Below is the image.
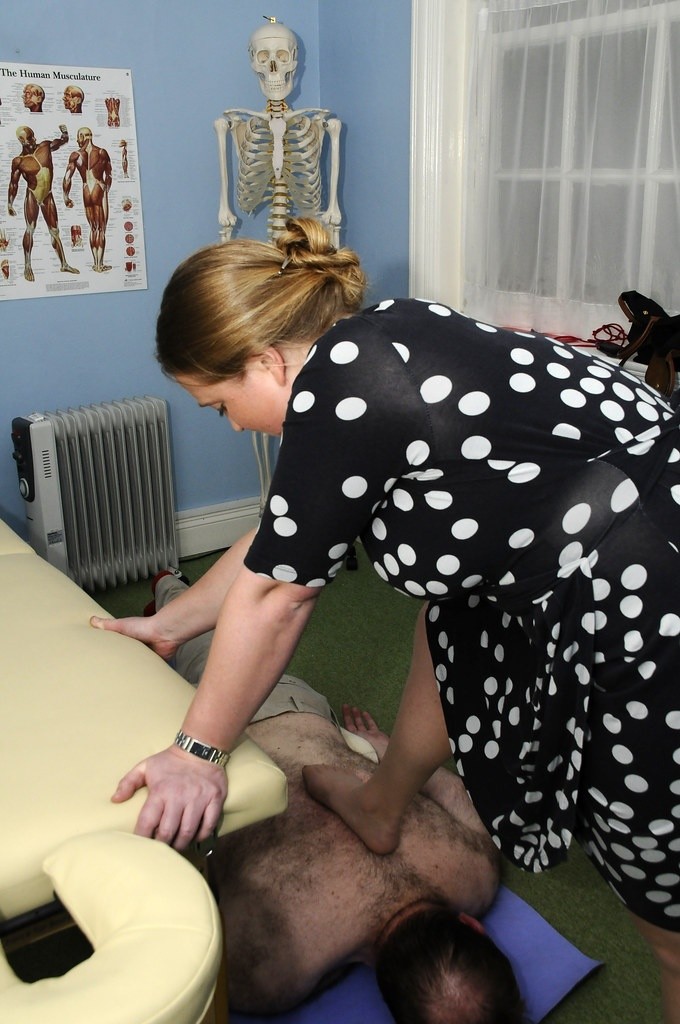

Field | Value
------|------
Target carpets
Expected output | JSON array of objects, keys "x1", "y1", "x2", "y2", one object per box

[{"x1": 227, "y1": 881, "x2": 603, "y2": 1024}]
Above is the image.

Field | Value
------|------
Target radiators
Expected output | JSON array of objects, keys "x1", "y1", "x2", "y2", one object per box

[{"x1": 11, "y1": 397, "x2": 184, "y2": 594}]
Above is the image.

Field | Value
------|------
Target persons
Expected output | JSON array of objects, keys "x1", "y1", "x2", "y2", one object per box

[{"x1": 96, "y1": 213, "x2": 679, "y2": 1021}]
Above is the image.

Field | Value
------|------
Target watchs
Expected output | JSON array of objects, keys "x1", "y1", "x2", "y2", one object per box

[{"x1": 174, "y1": 731, "x2": 231, "y2": 768}]
